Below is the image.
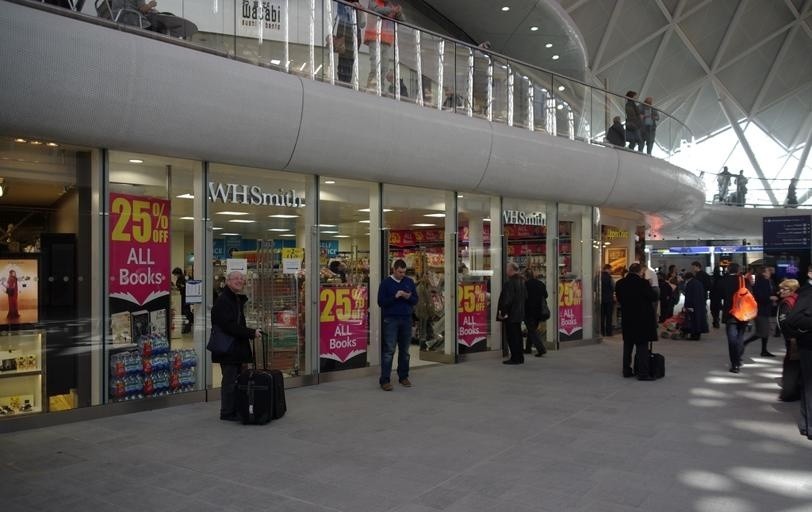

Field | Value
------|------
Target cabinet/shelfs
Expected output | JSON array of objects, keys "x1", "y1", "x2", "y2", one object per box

[
  {"x1": 225, "y1": 238, "x2": 574, "y2": 379},
  {"x1": 0, "y1": 328, "x2": 48, "y2": 418}
]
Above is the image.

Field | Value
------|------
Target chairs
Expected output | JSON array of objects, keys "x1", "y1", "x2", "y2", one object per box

[{"x1": 95, "y1": 0, "x2": 143, "y2": 29}]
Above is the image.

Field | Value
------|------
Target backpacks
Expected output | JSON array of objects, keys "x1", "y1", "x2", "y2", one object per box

[{"x1": 728, "y1": 275, "x2": 760, "y2": 322}]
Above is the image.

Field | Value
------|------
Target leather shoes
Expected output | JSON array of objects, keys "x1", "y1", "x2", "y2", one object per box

[
  {"x1": 686, "y1": 337, "x2": 700, "y2": 340},
  {"x1": 729, "y1": 365, "x2": 740, "y2": 373},
  {"x1": 762, "y1": 352, "x2": 774, "y2": 356},
  {"x1": 503, "y1": 348, "x2": 546, "y2": 364},
  {"x1": 624, "y1": 373, "x2": 634, "y2": 377},
  {"x1": 639, "y1": 375, "x2": 656, "y2": 381}
]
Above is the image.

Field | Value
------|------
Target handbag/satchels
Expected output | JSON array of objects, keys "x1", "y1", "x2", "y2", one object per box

[
  {"x1": 7, "y1": 288, "x2": 14, "y2": 295},
  {"x1": 682, "y1": 311, "x2": 697, "y2": 334},
  {"x1": 627, "y1": 119, "x2": 640, "y2": 130},
  {"x1": 540, "y1": 296, "x2": 551, "y2": 322},
  {"x1": 333, "y1": 36, "x2": 346, "y2": 54},
  {"x1": 207, "y1": 329, "x2": 237, "y2": 357},
  {"x1": 633, "y1": 350, "x2": 665, "y2": 378}
]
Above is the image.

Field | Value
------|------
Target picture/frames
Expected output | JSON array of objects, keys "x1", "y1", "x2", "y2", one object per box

[{"x1": 0, "y1": 251, "x2": 43, "y2": 331}]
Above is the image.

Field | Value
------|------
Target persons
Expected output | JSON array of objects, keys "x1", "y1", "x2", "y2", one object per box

[
  {"x1": 171, "y1": 268, "x2": 193, "y2": 334},
  {"x1": 6, "y1": 269, "x2": 20, "y2": 319},
  {"x1": 337, "y1": 0, "x2": 409, "y2": 101},
  {"x1": 522, "y1": 268, "x2": 548, "y2": 357},
  {"x1": 442, "y1": 84, "x2": 466, "y2": 110},
  {"x1": 496, "y1": 261, "x2": 528, "y2": 365},
  {"x1": 784, "y1": 178, "x2": 798, "y2": 208},
  {"x1": 716, "y1": 166, "x2": 738, "y2": 201},
  {"x1": 206, "y1": 270, "x2": 262, "y2": 422},
  {"x1": 377, "y1": 260, "x2": 419, "y2": 391},
  {"x1": 607, "y1": 90, "x2": 659, "y2": 154},
  {"x1": 733, "y1": 170, "x2": 748, "y2": 207},
  {"x1": 112, "y1": 0, "x2": 168, "y2": 34},
  {"x1": 329, "y1": 260, "x2": 346, "y2": 283},
  {"x1": 594, "y1": 262, "x2": 812, "y2": 440}
]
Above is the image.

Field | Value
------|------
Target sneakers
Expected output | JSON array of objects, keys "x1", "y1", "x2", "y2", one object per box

[
  {"x1": 381, "y1": 383, "x2": 393, "y2": 391},
  {"x1": 220, "y1": 413, "x2": 236, "y2": 420},
  {"x1": 399, "y1": 379, "x2": 412, "y2": 387}
]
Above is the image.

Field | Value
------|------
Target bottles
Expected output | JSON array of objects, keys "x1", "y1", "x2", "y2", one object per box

[{"x1": 110, "y1": 332, "x2": 197, "y2": 401}]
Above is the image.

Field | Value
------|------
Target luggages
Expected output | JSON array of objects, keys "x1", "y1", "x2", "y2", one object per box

[
  {"x1": 264, "y1": 333, "x2": 287, "y2": 419},
  {"x1": 235, "y1": 331, "x2": 272, "y2": 426}
]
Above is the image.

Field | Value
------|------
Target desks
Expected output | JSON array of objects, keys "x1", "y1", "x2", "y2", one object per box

[{"x1": 148, "y1": 13, "x2": 198, "y2": 39}]
